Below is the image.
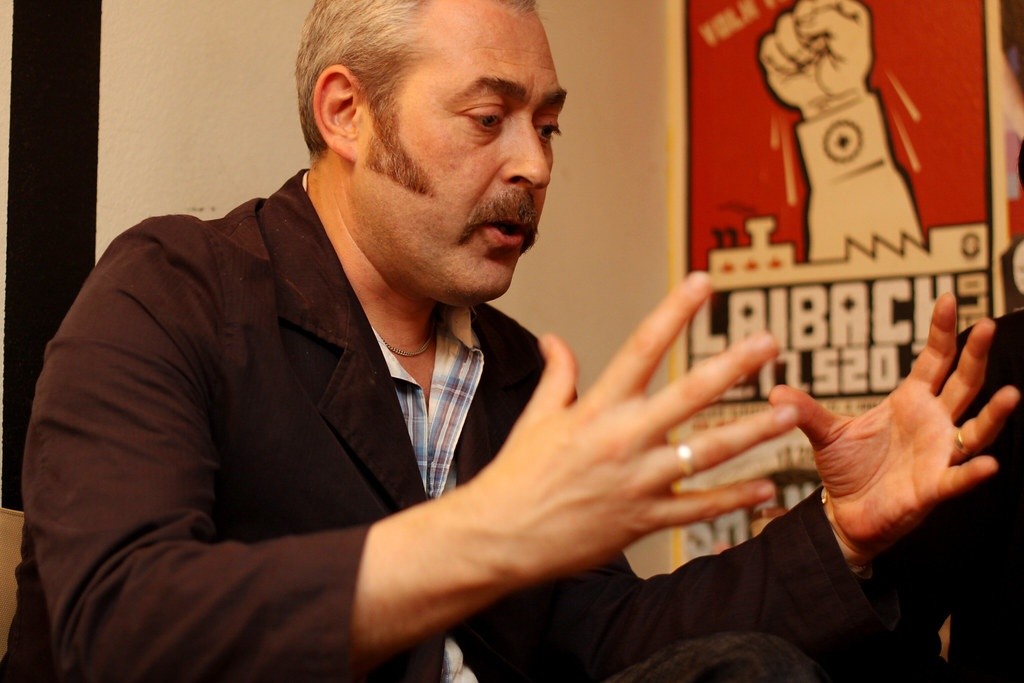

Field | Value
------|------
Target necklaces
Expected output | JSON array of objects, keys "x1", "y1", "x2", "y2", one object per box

[{"x1": 383, "y1": 320, "x2": 437, "y2": 355}]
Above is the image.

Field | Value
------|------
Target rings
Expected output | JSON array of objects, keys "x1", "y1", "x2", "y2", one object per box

[
  {"x1": 678, "y1": 443, "x2": 693, "y2": 477},
  {"x1": 956, "y1": 430, "x2": 970, "y2": 453}
]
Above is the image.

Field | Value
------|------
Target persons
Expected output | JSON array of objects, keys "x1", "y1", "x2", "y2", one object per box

[
  {"x1": 818, "y1": 145, "x2": 1024, "y2": 682},
  {"x1": 0, "y1": 0, "x2": 1019, "y2": 683}
]
